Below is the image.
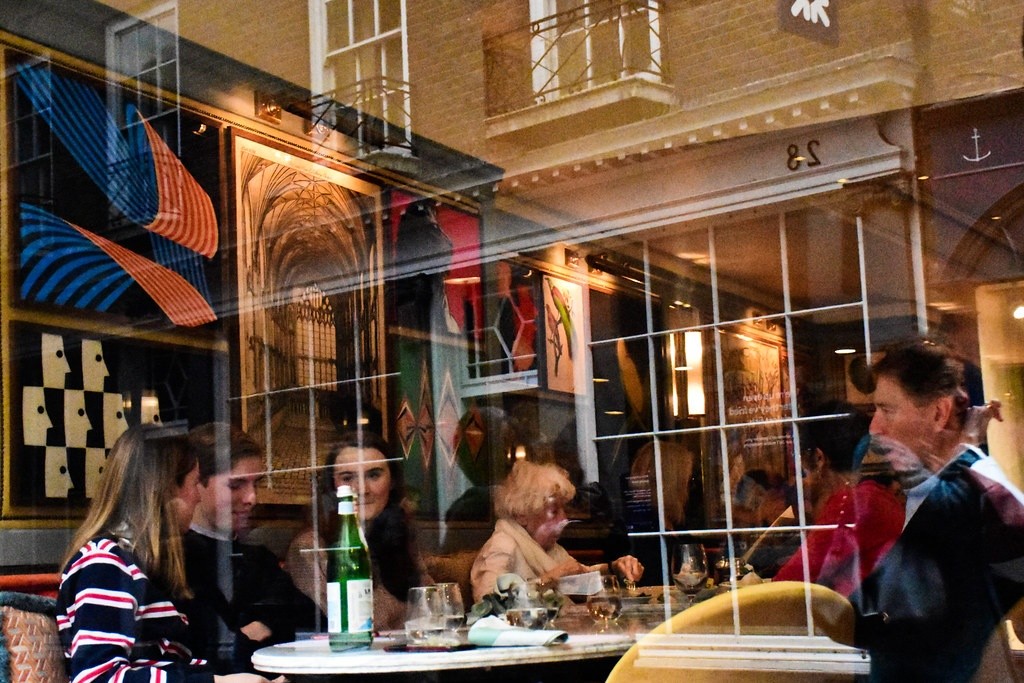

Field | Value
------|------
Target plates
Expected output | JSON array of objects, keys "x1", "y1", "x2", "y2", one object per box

[
  {"x1": 719, "y1": 581, "x2": 745, "y2": 587},
  {"x1": 621, "y1": 596, "x2": 653, "y2": 605}
]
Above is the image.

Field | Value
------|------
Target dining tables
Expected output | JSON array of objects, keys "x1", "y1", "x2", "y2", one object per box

[{"x1": 250, "y1": 630, "x2": 635, "y2": 683}]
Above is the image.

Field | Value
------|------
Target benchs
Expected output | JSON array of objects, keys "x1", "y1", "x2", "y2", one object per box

[{"x1": 1, "y1": 550, "x2": 605, "y2": 600}]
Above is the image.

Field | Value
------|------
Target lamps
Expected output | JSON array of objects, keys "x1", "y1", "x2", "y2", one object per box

[{"x1": 675, "y1": 330, "x2": 706, "y2": 417}]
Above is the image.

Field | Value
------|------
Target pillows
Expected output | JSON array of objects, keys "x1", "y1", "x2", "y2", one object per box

[
  {"x1": 0, "y1": 590, "x2": 68, "y2": 683},
  {"x1": 428, "y1": 553, "x2": 478, "y2": 613}
]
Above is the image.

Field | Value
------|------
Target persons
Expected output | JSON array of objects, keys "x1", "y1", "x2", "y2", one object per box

[
  {"x1": 52, "y1": 422, "x2": 287, "y2": 683},
  {"x1": 738, "y1": 395, "x2": 924, "y2": 607},
  {"x1": 469, "y1": 460, "x2": 644, "y2": 606},
  {"x1": 869, "y1": 472, "x2": 907, "y2": 513},
  {"x1": 175, "y1": 423, "x2": 339, "y2": 683},
  {"x1": 831, "y1": 341, "x2": 1023, "y2": 683},
  {"x1": 285, "y1": 427, "x2": 466, "y2": 639}
]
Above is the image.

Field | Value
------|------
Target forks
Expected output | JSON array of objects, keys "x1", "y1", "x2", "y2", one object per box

[{"x1": 626, "y1": 580, "x2": 635, "y2": 591}]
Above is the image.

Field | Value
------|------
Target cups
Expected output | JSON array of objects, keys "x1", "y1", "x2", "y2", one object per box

[
  {"x1": 587, "y1": 576, "x2": 622, "y2": 622},
  {"x1": 505, "y1": 583, "x2": 546, "y2": 630},
  {"x1": 405, "y1": 586, "x2": 447, "y2": 648},
  {"x1": 543, "y1": 588, "x2": 559, "y2": 628},
  {"x1": 724, "y1": 558, "x2": 744, "y2": 581},
  {"x1": 434, "y1": 582, "x2": 464, "y2": 647}
]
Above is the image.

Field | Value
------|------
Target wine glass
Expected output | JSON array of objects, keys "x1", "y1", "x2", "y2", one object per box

[{"x1": 671, "y1": 544, "x2": 708, "y2": 607}]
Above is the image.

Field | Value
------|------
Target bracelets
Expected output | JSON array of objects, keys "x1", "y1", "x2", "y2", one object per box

[{"x1": 607, "y1": 562, "x2": 615, "y2": 575}]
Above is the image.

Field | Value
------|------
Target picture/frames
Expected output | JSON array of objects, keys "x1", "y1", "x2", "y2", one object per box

[
  {"x1": 9, "y1": 319, "x2": 226, "y2": 506},
  {"x1": 227, "y1": 126, "x2": 398, "y2": 521},
  {"x1": 540, "y1": 271, "x2": 586, "y2": 398},
  {"x1": 496, "y1": 258, "x2": 545, "y2": 391},
  {"x1": 390, "y1": 186, "x2": 488, "y2": 342},
  {"x1": 587, "y1": 283, "x2": 668, "y2": 524},
  {"x1": 700, "y1": 328, "x2": 789, "y2": 527},
  {"x1": 390, "y1": 333, "x2": 495, "y2": 530},
  {"x1": 3, "y1": 48, "x2": 227, "y2": 340}
]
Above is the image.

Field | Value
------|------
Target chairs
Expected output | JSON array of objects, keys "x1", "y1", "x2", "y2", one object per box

[{"x1": 605, "y1": 581, "x2": 856, "y2": 683}]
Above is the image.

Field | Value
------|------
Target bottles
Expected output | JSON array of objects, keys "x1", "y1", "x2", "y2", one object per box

[
  {"x1": 335, "y1": 484, "x2": 373, "y2": 581},
  {"x1": 327, "y1": 501, "x2": 374, "y2": 652}
]
